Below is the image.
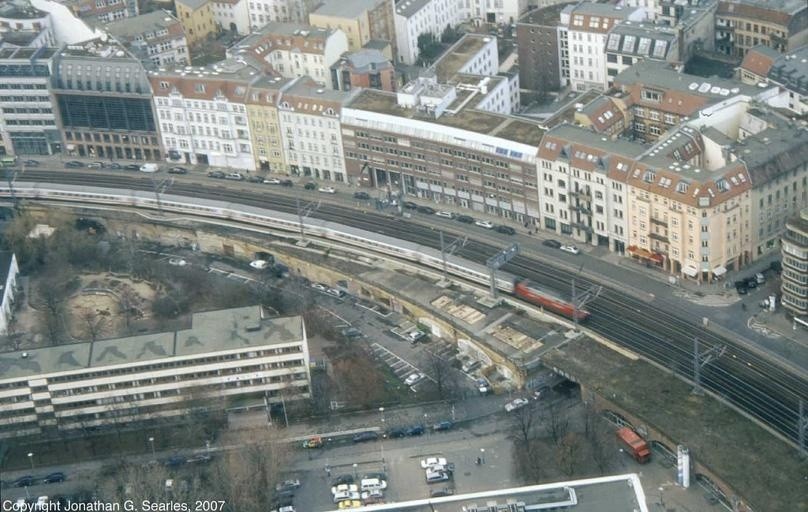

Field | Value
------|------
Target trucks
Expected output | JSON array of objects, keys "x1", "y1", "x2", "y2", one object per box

[{"x1": 617, "y1": 426, "x2": 650, "y2": 464}]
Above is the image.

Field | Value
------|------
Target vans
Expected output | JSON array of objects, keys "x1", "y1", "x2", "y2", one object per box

[
  {"x1": 302, "y1": 437, "x2": 322, "y2": 449},
  {"x1": 1, "y1": 157, "x2": 16, "y2": 167},
  {"x1": 248, "y1": 259, "x2": 346, "y2": 298},
  {"x1": 407, "y1": 330, "x2": 426, "y2": 343},
  {"x1": 734, "y1": 272, "x2": 766, "y2": 294}
]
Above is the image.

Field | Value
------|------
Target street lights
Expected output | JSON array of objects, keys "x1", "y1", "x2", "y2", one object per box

[
  {"x1": 27, "y1": 452, "x2": 34, "y2": 469},
  {"x1": 149, "y1": 437, "x2": 156, "y2": 456}
]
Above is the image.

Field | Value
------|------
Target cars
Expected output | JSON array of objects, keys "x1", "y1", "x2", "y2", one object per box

[
  {"x1": 354, "y1": 191, "x2": 515, "y2": 236},
  {"x1": 167, "y1": 166, "x2": 187, "y2": 174},
  {"x1": 462, "y1": 357, "x2": 492, "y2": 395},
  {"x1": 304, "y1": 180, "x2": 336, "y2": 194},
  {"x1": 421, "y1": 457, "x2": 453, "y2": 497},
  {"x1": 542, "y1": 239, "x2": 579, "y2": 255},
  {"x1": 0, "y1": 453, "x2": 213, "y2": 512},
  {"x1": 208, "y1": 170, "x2": 292, "y2": 186},
  {"x1": 505, "y1": 386, "x2": 549, "y2": 413},
  {"x1": 351, "y1": 420, "x2": 453, "y2": 443},
  {"x1": 64, "y1": 160, "x2": 159, "y2": 173},
  {"x1": 330, "y1": 472, "x2": 388, "y2": 509},
  {"x1": 405, "y1": 372, "x2": 425, "y2": 385},
  {"x1": 23, "y1": 160, "x2": 39, "y2": 167},
  {"x1": 269, "y1": 477, "x2": 300, "y2": 512}
]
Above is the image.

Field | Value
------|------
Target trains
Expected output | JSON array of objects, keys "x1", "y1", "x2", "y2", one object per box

[{"x1": 0, "y1": 180, "x2": 590, "y2": 322}]
